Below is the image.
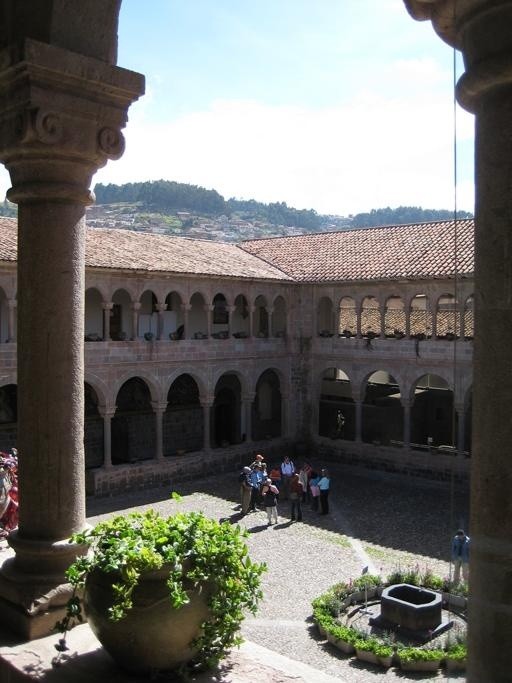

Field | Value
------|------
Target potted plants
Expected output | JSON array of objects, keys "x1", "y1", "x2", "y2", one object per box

[
  {"x1": 310, "y1": 568, "x2": 468, "y2": 672},
  {"x1": 48, "y1": 492, "x2": 270, "y2": 683}
]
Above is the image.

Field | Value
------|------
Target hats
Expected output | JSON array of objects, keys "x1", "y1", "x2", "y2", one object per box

[{"x1": 256, "y1": 455, "x2": 264, "y2": 460}]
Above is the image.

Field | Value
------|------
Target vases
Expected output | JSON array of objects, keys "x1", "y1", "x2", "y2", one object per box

[{"x1": 0, "y1": 465, "x2": 12, "y2": 519}]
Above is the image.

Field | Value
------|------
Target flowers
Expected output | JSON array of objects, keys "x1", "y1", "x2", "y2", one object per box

[{"x1": 0, "y1": 447, "x2": 20, "y2": 538}]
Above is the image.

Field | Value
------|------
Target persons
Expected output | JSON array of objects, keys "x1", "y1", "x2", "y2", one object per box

[
  {"x1": 452, "y1": 530, "x2": 470, "y2": 583},
  {"x1": 337, "y1": 409, "x2": 345, "y2": 439},
  {"x1": 240, "y1": 453, "x2": 329, "y2": 525}
]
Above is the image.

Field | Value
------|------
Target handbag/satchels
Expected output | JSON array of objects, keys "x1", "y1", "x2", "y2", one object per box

[
  {"x1": 290, "y1": 492, "x2": 297, "y2": 500},
  {"x1": 310, "y1": 485, "x2": 320, "y2": 497}
]
